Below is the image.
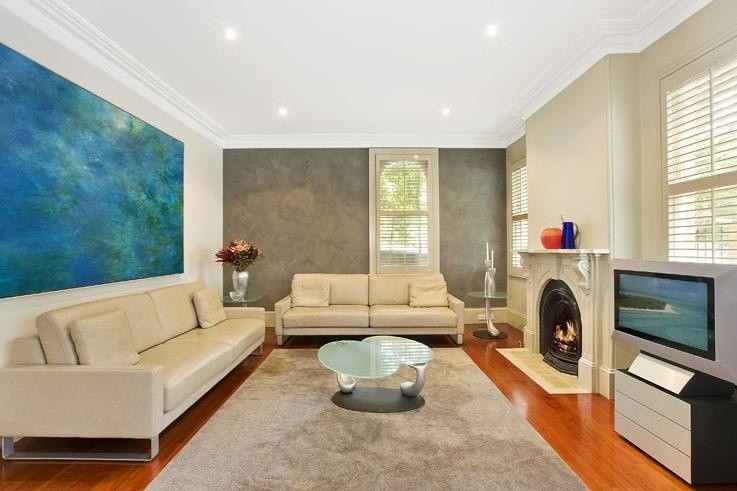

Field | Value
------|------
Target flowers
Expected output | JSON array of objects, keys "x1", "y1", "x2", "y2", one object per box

[{"x1": 213, "y1": 237, "x2": 262, "y2": 270}]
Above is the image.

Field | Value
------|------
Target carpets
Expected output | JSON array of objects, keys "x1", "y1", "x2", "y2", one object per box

[{"x1": 142, "y1": 343, "x2": 589, "y2": 490}]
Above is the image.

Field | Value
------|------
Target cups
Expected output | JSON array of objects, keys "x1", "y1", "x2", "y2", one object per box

[
  {"x1": 484, "y1": 260, "x2": 496, "y2": 298},
  {"x1": 560, "y1": 222, "x2": 578, "y2": 249},
  {"x1": 229, "y1": 292, "x2": 242, "y2": 301}
]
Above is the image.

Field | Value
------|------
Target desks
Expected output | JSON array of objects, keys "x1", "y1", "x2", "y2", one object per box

[{"x1": 608, "y1": 368, "x2": 736, "y2": 486}]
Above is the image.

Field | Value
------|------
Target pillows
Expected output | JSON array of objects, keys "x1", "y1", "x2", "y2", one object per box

[
  {"x1": 288, "y1": 276, "x2": 331, "y2": 309},
  {"x1": 408, "y1": 279, "x2": 448, "y2": 309},
  {"x1": 191, "y1": 287, "x2": 226, "y2": 330},
  {"x1": 68, "y1": 310, "x2": 139, "y2": 371}
]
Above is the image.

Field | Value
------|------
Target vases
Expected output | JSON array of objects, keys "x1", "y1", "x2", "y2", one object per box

[
  {"x1": 229, "y1": 269, "x2": 248, "y2": 298},
  {"x1": 540, "y1": 228, "x2": 562, "y2": 250}
]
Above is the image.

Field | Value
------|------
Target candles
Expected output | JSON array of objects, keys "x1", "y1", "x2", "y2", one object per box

[
  {"x1": 491, "y1": 250, "x2": 493, "y2": 270},
  {"x1": 486, "y1": 242, "x2": 488, "y2": 261}
]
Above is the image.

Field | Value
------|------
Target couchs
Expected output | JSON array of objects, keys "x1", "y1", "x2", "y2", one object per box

[
  {"x1": 269, "y1": 273, "x2": 465, "y2": 349},
  {"x1": 0, "y1": 271, "x2": 267, "y2": 466}
]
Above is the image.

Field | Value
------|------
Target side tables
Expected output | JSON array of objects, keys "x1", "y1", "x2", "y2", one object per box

[
  {"x1": 218, "y1": 293, "x2": 266, "y2": 308},
  {"x1": 467, "y1": 289, "x2": 514, "y2": 340}
]
